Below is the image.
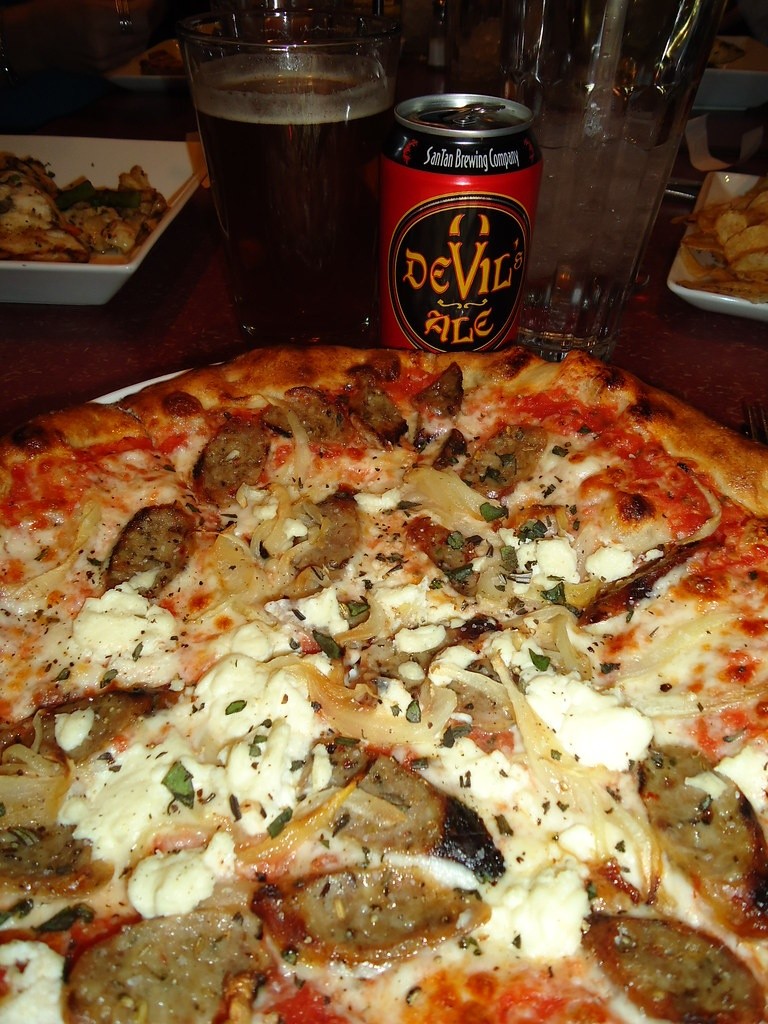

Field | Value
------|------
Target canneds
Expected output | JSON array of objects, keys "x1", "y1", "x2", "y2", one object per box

[{"x1": 382, "y1": 93, "x2": 540, "y2": 347}]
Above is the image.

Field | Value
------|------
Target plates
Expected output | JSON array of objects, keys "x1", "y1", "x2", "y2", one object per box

[
  {"x1": 0, "y1": 134, "x2": 213, "y2": 306},
  {"x1": 666, "y1": 171, "x2": 768, "y2": 322},
  {"x1": 693, "y1": 35, "x2": 768, "y2": 110},
  {"x1": 102, "y1": 38, "x2": 189, "y2": 93}
]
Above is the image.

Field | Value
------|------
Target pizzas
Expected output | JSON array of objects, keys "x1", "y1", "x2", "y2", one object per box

[{"x1": 0, "y1": 349, "x2": 768, "y2": 1024}]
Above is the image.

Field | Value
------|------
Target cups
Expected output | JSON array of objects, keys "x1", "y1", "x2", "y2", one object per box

[
  {"x1": 173, "y1": 8, "x2": 402, "y2": 351},
  {"x1": 497, "y1": 0, "x2": 725, "y2": 367}
]
